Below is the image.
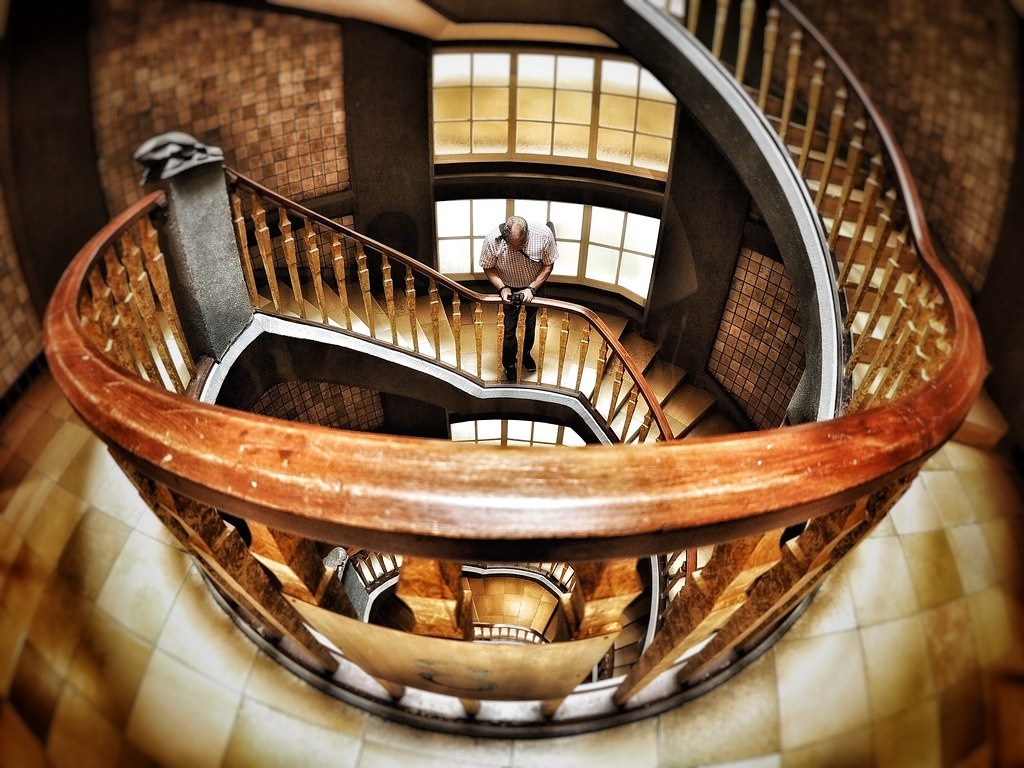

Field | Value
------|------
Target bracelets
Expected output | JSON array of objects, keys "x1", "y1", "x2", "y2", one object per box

[
  {"x1": 528, "y1": 287, "x2": 536, "y2": 294},
  {"x1": 499, "y1": 286, "x2": 506, "y2": 292}
]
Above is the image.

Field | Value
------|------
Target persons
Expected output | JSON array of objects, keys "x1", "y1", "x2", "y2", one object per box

[{"x1": 479, "y1": 216, "x2": 561, "y2": 383}]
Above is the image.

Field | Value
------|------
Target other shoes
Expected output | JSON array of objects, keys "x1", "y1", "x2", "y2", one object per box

[
  {"x1": 522, "y1": 354, "x2": 536, "y2": 371},
  {"x1": 505, "y1": 355, "x2": 516, "y2": 381}
]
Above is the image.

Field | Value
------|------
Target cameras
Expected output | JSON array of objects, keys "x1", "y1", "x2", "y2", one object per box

[{"x1": 508, "y1": 292, "x2": 525, "y2": 305}]
